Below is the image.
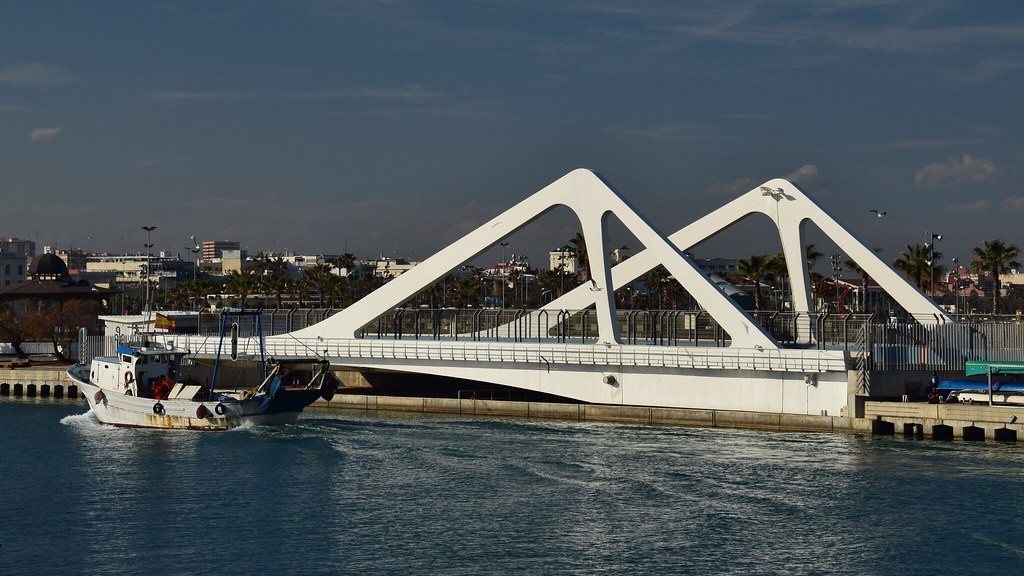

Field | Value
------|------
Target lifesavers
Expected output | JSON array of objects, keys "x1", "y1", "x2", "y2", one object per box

[
  {"x1": 153, "y1": 402, "x2": 163, "y2": 413},
  {"x1": 123, "y1": 371, "x2": 133, "y2": 384},
  {"x1": 215, "y1": 403, "x2": 226, "y2": 415}
]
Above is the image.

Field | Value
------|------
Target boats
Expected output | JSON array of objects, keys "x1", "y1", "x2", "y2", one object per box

[{"x1": 65, "y1": 284, "x2": 346, "y2": 433}]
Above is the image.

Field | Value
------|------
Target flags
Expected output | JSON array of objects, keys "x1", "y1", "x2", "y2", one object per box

[{"x1": 154, "y1": 312, "x2": 176, "y2": 332}]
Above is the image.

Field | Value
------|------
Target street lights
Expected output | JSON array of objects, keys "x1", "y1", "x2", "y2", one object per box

[
  {"x1": 499, "y1": 242, "x2": 509, "y2": 324},
  {"x1": 561, "y1": 245, "x2": 567, "y2": 295},
  {"x1": 191, "y1": 249, "x2": 201, "y2": 311},
  {"x1": 141, "y1": 226, "x2": 157, "y2": 316}
]
